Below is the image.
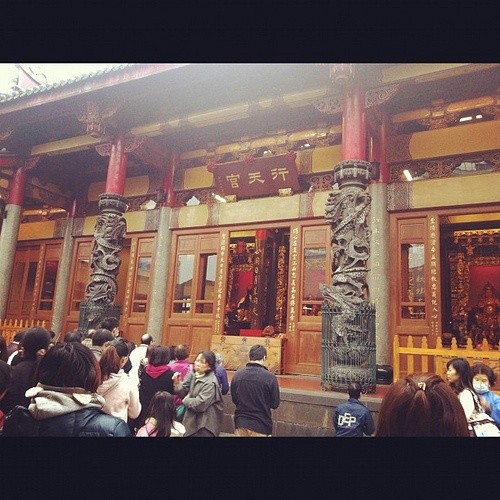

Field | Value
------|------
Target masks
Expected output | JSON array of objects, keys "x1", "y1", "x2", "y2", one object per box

[
  {"x1": 115, "y1": 330, "x2": 119, "y2": 336},
  {"x1": 472, "y1": 381, "x2": 489, "y2": 394}
]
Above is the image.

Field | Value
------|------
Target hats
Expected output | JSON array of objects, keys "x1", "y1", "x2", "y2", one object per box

[{"x1": 24, "y1": 327, "x2": 51, "y2": 357}]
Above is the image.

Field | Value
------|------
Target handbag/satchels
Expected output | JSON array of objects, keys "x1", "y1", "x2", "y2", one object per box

[
  {"x1": 468, "y1": 410, "x2": 500, "y2": 437},
  {"x1": 176, "y1": 404, "x2": 185, "y2": 416}
]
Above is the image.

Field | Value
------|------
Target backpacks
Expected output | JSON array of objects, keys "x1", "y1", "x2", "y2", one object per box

[{"x1": 3, "y1": 405, "x2": 36, "y2": 436}]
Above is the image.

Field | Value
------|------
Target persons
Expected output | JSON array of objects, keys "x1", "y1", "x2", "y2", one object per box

[
  {"x1": 332, "y1": 383, "x2": 375, "y2": 437},
  {"x1": 375, "y1": 372, "x2": 469, "y2": 437},
  {"x1": 446, "y1": 358, "x2": 500, "y2": 437},
  {"x1": 0, "y1": 316, "x2": 229, "y2": 437},
  {"x1": 230, "y1": 345, "x2": 280, "y2": 437}
]
